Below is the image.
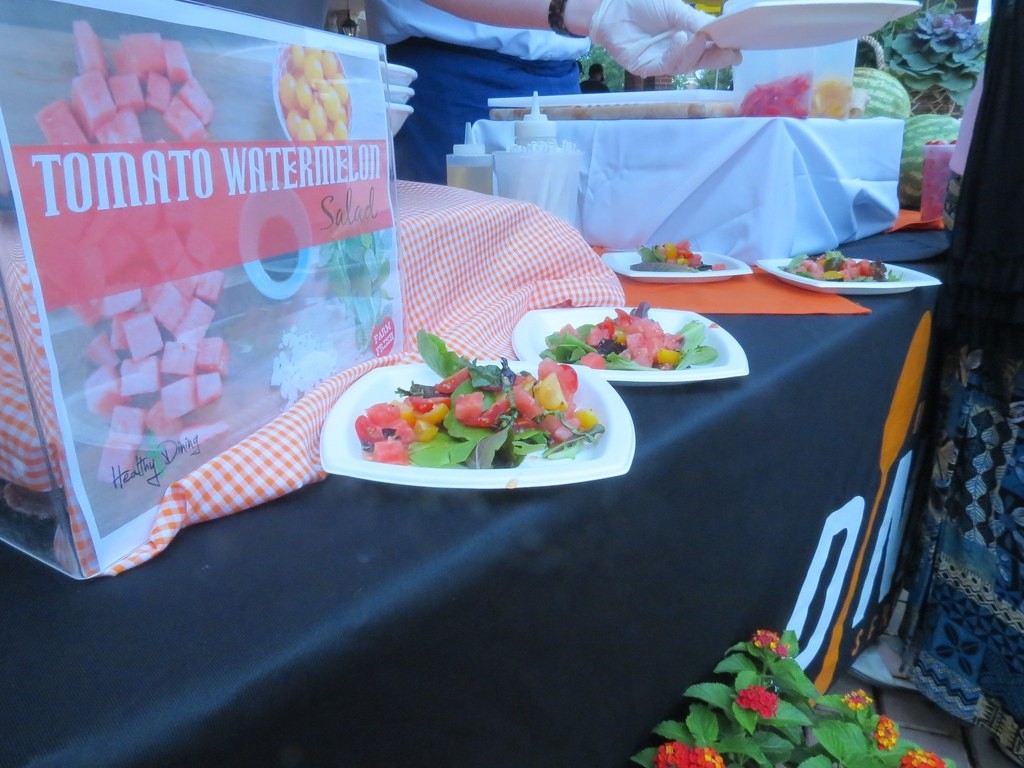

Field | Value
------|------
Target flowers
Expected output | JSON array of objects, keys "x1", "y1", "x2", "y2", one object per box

[{"x1": 629, "y1": 629, "x2": 957, "y2": 768}]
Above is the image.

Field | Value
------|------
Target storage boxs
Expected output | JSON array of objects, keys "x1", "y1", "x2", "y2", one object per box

[{"x1": 921, "y1": 144, "x2": 954, "y2": 216}]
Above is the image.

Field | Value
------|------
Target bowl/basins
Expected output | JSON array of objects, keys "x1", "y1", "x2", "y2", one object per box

[{"x1": 381, "y1": 62, "x2": 418, "y2": 136}]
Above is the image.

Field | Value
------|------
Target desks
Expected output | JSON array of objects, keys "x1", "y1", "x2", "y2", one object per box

[{"x1": 0, "y1": 219, "x2": 949, "y2": 768}]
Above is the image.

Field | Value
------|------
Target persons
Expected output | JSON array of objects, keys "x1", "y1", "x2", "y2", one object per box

[
  {"x1": 198, "y1": 0, "x2": 743, "y2": 185},
  {"x1": 893, "y1": 0, "x2": 1023, "y2": 755}
]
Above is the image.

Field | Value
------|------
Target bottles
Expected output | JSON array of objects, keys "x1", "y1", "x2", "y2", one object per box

[
  {"x1": 514, "y1": 91, "x2": 557, "y2": 151},
  {"x1": 446, "y1": 122, "x2": 493, "y2": 195}
]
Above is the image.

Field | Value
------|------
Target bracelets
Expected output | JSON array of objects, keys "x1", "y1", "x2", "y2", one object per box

[{"x1": 547, "y1": 0, "x2": 588, "y2": 40}]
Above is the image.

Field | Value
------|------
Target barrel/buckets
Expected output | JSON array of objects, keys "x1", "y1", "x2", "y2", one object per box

[{"x1": 723, "y1": 0, "x2": 856, "y2": 118}]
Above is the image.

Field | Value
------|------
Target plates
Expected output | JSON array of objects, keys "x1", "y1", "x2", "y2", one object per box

[
  {"x1": 756, "y1": 258, "x2": 942, "y2": 294},
  {"x1": 512, "y1": 307, "x2": 749, "y2": 385},
  {"x1": 320, "y1": 360, "x2": 636, "y2": 488},
  {"x1": 602, "y1": 251, "x2": 753, "y2": 283},
  {"x1": 696, "y1": 0, "x2": 921, "y2": 49},
  {"x1": 273, "y1": 43, "x2": 353, "y2": 140}
]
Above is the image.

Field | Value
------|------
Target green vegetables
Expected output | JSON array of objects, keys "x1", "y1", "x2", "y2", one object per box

[
  {"x1": 628, "y1": 245, "x2": 713, "y2": 274},
  {"x1": 392, "y1": 329, "x2": 605, "y2": 470},
  {"x1": 538, "y1": 301, "x2": 717, "y2": 372}
]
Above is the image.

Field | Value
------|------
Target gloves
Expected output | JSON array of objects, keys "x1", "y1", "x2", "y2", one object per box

[{"x1": 590, "y1": 0, "x2": 743, "y2": 78}]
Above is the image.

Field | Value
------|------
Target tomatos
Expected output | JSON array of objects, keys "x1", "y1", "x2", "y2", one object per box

[
  {"x1": 409, "y1": 396, "x2": 451, "y2": 412},
  {"x1": 922, "y1": 139, "x2": 958, "y2": 221},
  {"x1": 859, "y1": 260, "x2": 874, "y2": 276}
]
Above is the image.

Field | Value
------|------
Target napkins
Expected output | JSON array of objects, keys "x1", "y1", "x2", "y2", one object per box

[
  {"x1": 593, "y1": 245, "x2": 872, "y2": 315},
  {"x1": 885, "y1": 209, "x2": 945, "y2": 233}
]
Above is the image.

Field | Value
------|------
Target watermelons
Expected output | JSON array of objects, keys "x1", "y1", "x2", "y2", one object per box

[
  {"x1": 34, "y1": 20, "x2": 231, "y2": 484},
  {"x1": 674, "y1": 240, "x2": 725, "y2": 270},
  {"x1": 845, "y1": 66, "x2": 911, "y2": 123},
  {"x1": 897, "y1": 113, "x2": 963, "y2": 209},
  {"x1": 353, "y1": 357, "x2": 581, "y2": 464},
  {"x1": 559, "y1": 317, "x2": 684, "y2": 370},
  {"x1": 797, "y1": 253, "x2": 860, "y2": 281}
]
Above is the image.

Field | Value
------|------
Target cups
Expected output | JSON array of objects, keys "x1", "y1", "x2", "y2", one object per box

[
  {"x1": 494, "y1": 151, "x2": 581, "y2": 224},
  {"x1": 920, "y1": 145, "x2": 956, "y2": 227},
  {"x1": 813, "y1": 39, "x2": 858, "y2": 118}
]
